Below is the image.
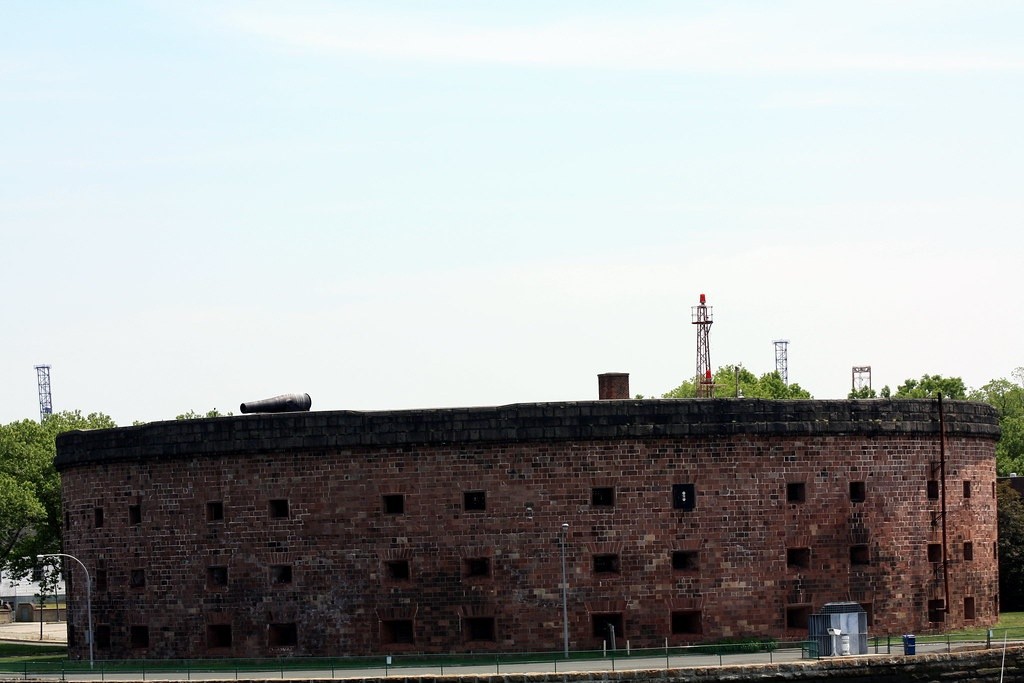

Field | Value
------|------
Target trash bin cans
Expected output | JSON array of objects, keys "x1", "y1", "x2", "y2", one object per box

[{"x1": 902, "y1": 633, "x2": 917, "y2": 656}]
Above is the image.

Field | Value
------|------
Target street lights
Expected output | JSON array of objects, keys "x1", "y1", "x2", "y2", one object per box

[
  {"x1": 561, "y1": 523, "x2": 570, "y2": 661},
  {"x1": 36, "y1": 553, "x2": 95, "y2": 673}
]
